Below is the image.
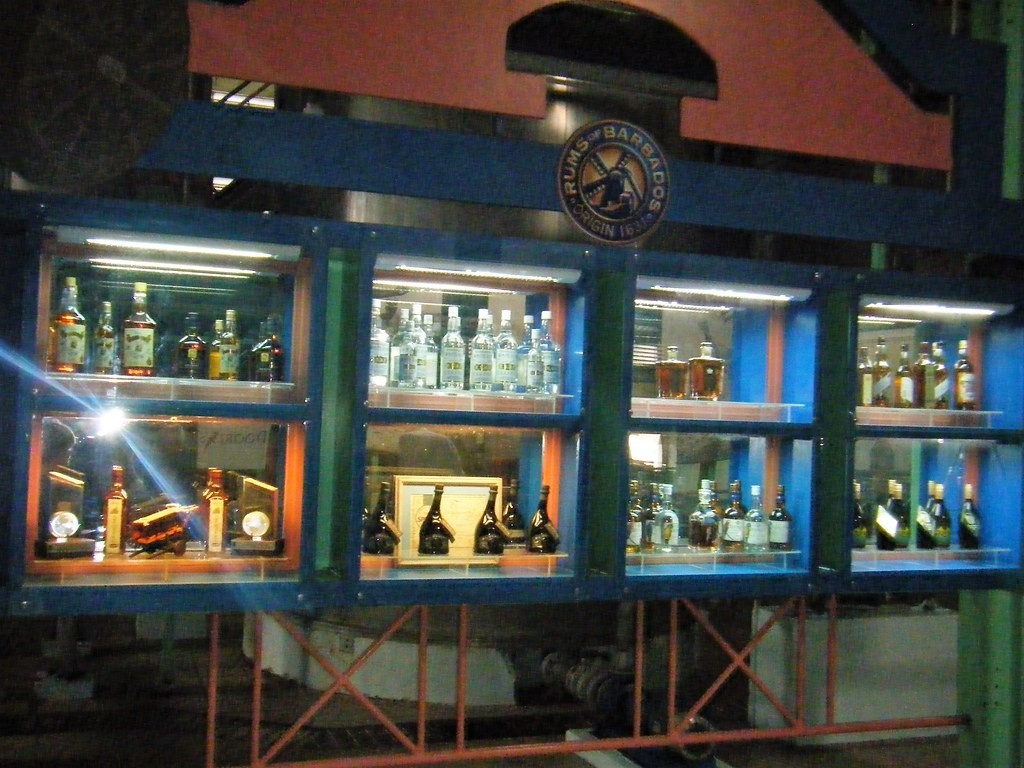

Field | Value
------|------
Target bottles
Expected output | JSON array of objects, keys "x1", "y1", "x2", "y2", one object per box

[
  {"x1": 654, "y1": 346, "x2": 688, "y2": 398},
  {"x1": 687, "y1": 341, "x2": 725, "y2": 400},
  {"x1": 626, "y1": 478, "x2": 792, "y2": 554},
  {"x1": 101, "y1": 465, "x2": 129, "y2": 555},
  {"x1": 202, "y1": 468, "x2": 228, "y2": 554},
  {"x1": 369, "y1": 297, "x2": 561, "y2": 394},
  {"x1": 177, "y1": 308, "x2": 285, "y2": 384},
  {"x1": 954, "y1": 340, "x2": 976, "y2": 410},
  {"x1": 46, "y1": 277, "x2": 87, "y2": 374},
  {"x1": 857, "y1": 335, "x2": 949, "y2": 409},
  {"x1": 364, "y1": 482, "x2": 394, "y2": 555},
  {"x1": 526, "y1": 486, "x2": 558, "y2": 554},
  {"x1": 119, "y1": 281, "x2": 156, "y2": 377},
  {"x1": 502, "y1": 479, "x2": 526, "y2": 544},
  {"x1": 91, "y1": 301, "x2": 115, "y2": 375},
  {"x1": 418, "y1": 483, "x2": 449, "y2": 555},
  {"x1": 474, "y1": 485, "x2": 503, "y2": 554},
  {"x1": 853, "y1": 478, "x2": 980, "y2": 549}
]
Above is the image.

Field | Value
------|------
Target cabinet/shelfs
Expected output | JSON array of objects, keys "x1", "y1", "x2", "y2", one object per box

[{"x1": 0, "y1": 190, "x2": 1024, "y2": 616}]
